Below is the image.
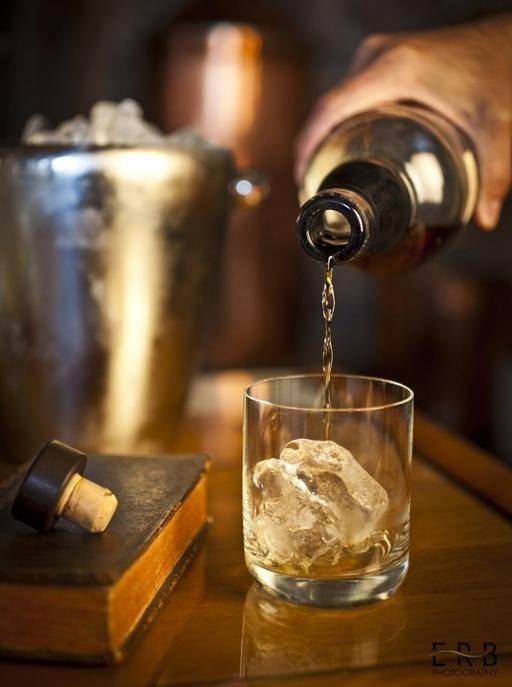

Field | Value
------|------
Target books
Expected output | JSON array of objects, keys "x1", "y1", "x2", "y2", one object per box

[{"x1": 1, "y1": 444, "x2": 216, "y2": 666}]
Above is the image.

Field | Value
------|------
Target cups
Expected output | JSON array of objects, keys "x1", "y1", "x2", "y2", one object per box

[{"x1": 241, "y1": 374, "x2": 414, "y2": 609}]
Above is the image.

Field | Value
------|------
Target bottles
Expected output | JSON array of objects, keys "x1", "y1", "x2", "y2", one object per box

[{"x1": 294, "y1": 102, "x2": 482, "y2": 282}]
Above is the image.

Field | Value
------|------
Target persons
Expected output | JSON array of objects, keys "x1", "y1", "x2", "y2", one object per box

[{"x1": 293, "y1": 8, "x2": 511, "y2": 236}]
